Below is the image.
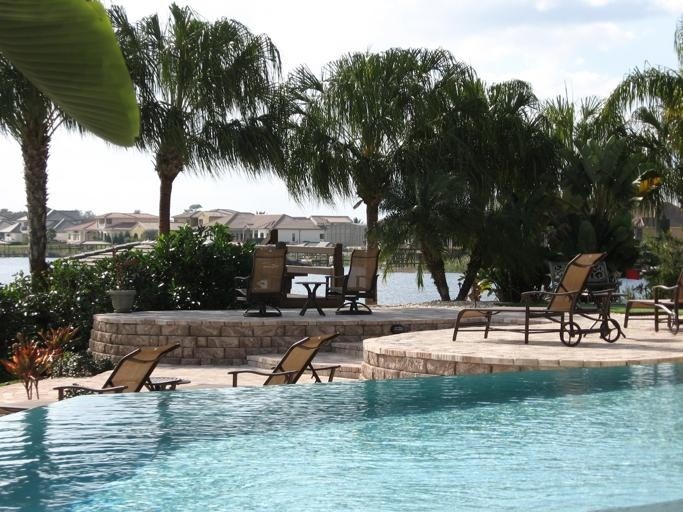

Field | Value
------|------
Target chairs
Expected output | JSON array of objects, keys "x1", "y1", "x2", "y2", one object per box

[
  {"x1": 53, "y1": 343, "x2": 182, "y2": 400},
  {"x1": 227, "y1": 333, "x2": 342, "y2": 387},
  {"x1": 453, "y1": 252, "x2": 683, "y2": 347},
  {"x1": 325, "y1": 248, "x2": 381, "y2": 315},
  {"x1": 233, "y1": 245, "x2": 288, "y2": 317}
]
